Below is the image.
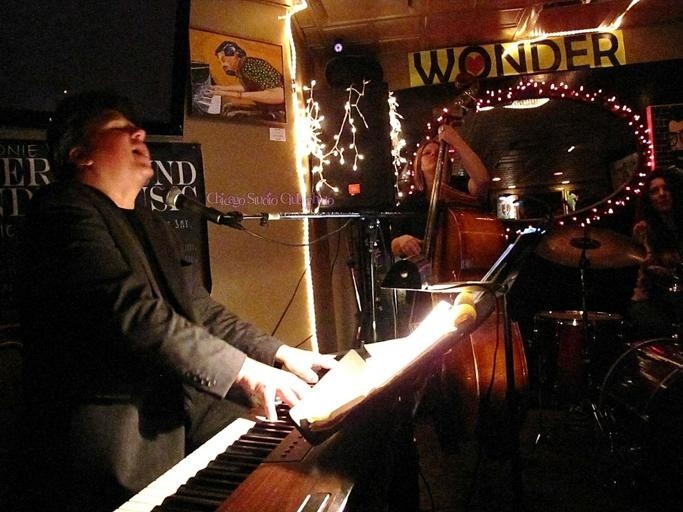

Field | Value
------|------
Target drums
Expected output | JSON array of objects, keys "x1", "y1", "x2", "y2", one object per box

[
  {"x1": 594, "y1": 336, "x2": 682, "y2": 464},
  {"x1": 536, "y1": 309, "x2": 619, "y2": 383}
]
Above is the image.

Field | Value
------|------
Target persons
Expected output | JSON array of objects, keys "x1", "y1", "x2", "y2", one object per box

[
  {"x1": 666, "y1": 108, "x2": 683, "y2": 174},
  {"x1": 202, "y1": 40, "x2": 286, "y2": 125},
  {"x1": 12, "y1": 91, "x2": 340, "y2": 511},
  {"x1": 387, "y1": 123, "x2": 492, "y2": 264},
  {"x1": 632, "y1": 166, "x2": 683, "y2": 266}
]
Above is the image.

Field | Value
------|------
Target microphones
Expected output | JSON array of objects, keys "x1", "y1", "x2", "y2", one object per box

[{"x1": 162, "y1": 186, "x2": 247, "y2": 232}]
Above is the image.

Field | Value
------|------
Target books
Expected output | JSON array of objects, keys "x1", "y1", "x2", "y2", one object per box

[{"x1": 307, "y1": 301, "x2": 459, "y2": 434}]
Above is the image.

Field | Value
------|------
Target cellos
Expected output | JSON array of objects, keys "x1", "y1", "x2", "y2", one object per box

[{"x1": 391, "y1": 73, "x2": 531, "y2": 407}]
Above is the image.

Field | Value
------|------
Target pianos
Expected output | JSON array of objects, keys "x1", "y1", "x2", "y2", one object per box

[
  {"x1": 190, "y1": 62, "x2": 221, "y2": 118},
  {"x1": 112, "y1": 354, "x2": 420, "y2": 512}
]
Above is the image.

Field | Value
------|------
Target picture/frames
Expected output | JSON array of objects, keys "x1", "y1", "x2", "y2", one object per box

[{"x1": 187, "y1": 24, "x2": 290, "y2": 129}]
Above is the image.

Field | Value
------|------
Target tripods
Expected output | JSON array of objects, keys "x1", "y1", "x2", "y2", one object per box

[{"x1": 532, "y1": 252, "x2": 610, "y2": 448}]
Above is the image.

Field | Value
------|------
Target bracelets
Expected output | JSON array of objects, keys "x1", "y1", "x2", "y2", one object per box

[{"x1": 234, "y1": 90, "x2": 242, "y2": 99}]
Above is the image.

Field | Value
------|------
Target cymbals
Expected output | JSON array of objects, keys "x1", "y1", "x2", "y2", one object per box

[{"x1": 534, "y1": 222, "x2": 646, "y2": 271}]
